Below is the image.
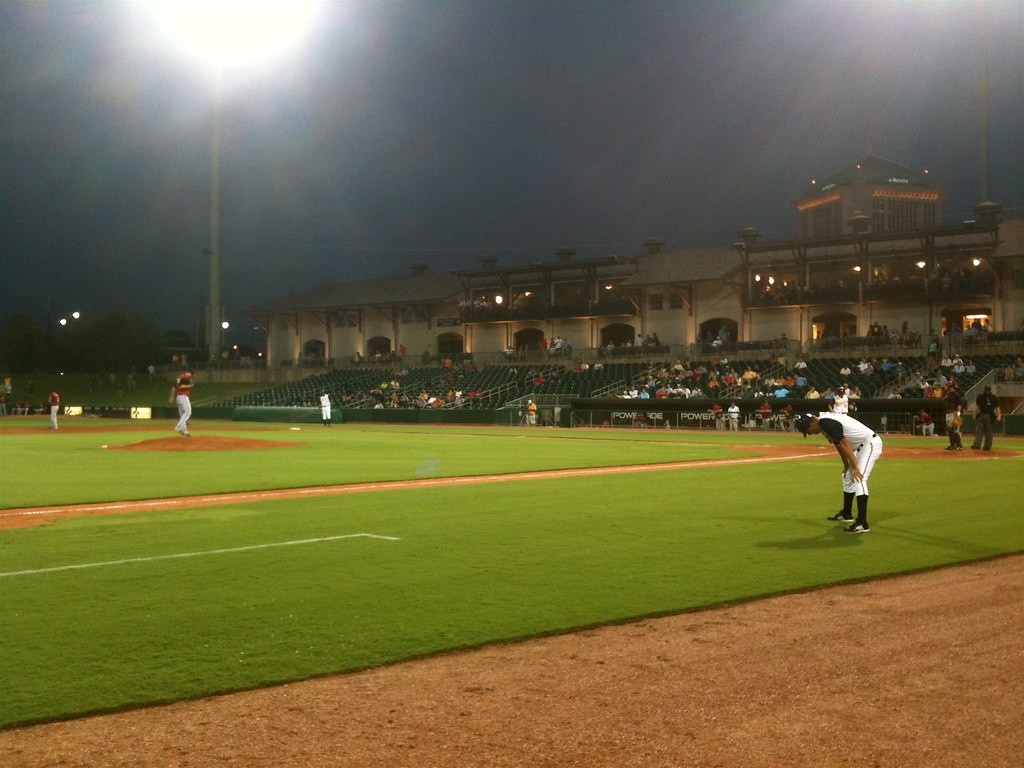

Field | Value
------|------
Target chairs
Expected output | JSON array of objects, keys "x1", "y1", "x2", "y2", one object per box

[{"x1": 211, "y1": 356, "x2": 1020, "y2": 408}]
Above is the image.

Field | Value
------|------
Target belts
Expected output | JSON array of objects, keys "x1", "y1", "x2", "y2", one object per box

[{"x1": 857, "y1": 433, "x2": 877, "y2": 452}]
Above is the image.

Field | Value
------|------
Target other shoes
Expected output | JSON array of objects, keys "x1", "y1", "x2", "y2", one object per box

[
  {"x1": 945, "y1": 446, "x2": 955, "y2": 450},
  {"x1": 971, "y1": 445, "x2": 980, "y2": 449},
  {"x1": 983, "y1": 447, "x2": 989, "y2": 450},
  {"x1": 956, "y1": 446, "x2": 963, "y2": 450}
]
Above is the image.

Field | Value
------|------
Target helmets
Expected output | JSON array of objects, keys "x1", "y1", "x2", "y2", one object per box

[
  {"x1": 984, "y1": 385, "x2": 992, "y2": 390},
  {"x1": 944, "y1": 381, "x2": 955, "y2": 386},
  {"x1": 794, "y1": 412, "x2": 812, "y2": 437}
]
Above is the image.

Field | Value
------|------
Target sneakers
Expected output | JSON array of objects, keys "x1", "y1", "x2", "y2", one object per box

[
  {"x1": 842, "y1": 523, "x2": 870, "y2": 534},
  {"x1": 827, "y1": 511, "x2": 853, "y2": 522}
]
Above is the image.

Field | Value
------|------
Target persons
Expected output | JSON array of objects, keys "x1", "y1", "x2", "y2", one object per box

[
  {"x1": 0, "y1": 376, "x2": 47, "y2": 418},
  {"x1": 811, "y1": 317, "x2": 992, "y2": 376},
  {"x1": 48, "y1": 389, "x2": 60, "y2": 431},
  {"x1": 944, "y1": 380, "x2": 962, "y2": 451},
  {"x1": 146, "y1": 345, "x2": 187, "y2": 381},
  {"x1": 828, "y1": 389, "x2": 857, "y2": 416},
  {"x1": 916, "y1": 409, "x2": 938, "y2": 438},
  {"x1": 971, "y1": 385, "x2": 1001, "y2": 451},
  {"x1": 1002, "y1": 357, "x2": 1024, "y2": 383},
  {"x1": 286, "y1": 324, "x2": 812, "y2": 432},
  {"x1": 887, "y1": 353, "x2": 976, "y2": 399},
  {"x1": 794, "y1": 412, "x2": 883, "y2": 534},
  {"x1": 804, "y1": 383, "x2": 862, "y2": 399},
  {"x1": 169, "y1": 370, "x2": 196, "y2": 437}
]
